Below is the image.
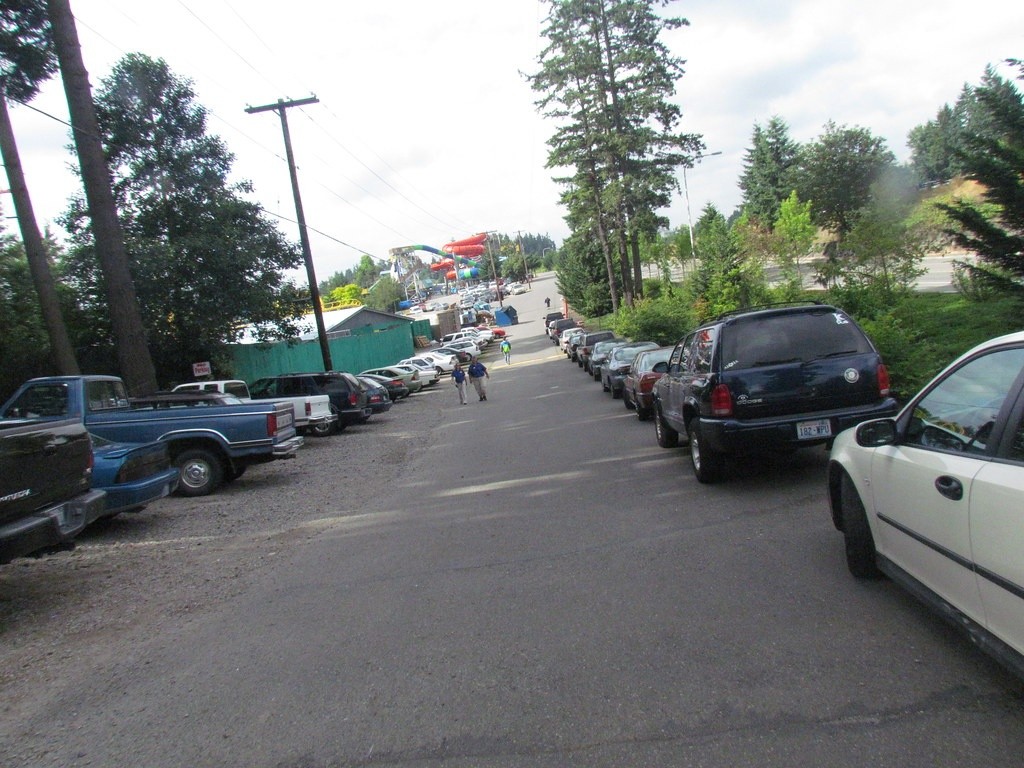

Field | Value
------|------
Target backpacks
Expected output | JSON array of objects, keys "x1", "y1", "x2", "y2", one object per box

[{"x1": 502, "y1": 342, "x2": 510, "y2": 353}]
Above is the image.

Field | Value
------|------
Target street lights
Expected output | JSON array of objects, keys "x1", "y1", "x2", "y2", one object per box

[{"x1": 684, "y1": 151, "x2": 723, "y2": 280}]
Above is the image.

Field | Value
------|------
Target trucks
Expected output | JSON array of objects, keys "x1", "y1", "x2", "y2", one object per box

[
  {"x1": 0, "y1": 376, "x2": 304, "y2": 494},
  {"x1": 165, "y1": 379, "x2": 340, "y2": 440},
  {"x1": 0, "y1": 419, "x2": 107, "y2": 565}
]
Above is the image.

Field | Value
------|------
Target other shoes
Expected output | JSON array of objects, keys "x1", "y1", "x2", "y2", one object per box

[
  {"x1": 482, "y1": 394, "x2": 487, "y2": 401},
  {"x1": 479, "y1": 396, "x2": 483, "y2": 402}
]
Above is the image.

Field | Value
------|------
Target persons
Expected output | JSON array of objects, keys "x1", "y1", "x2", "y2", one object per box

[
  {"x1": 468, "y1": 355, "x2": 490, "y2": 402},
  {"x1": 544, "y1": 297, "x2": 552, "y2": 306},
  {"x1": 452, "y1": 363, "x2": 469, "y2": 405},
  {"x1": 499, "y1": 336, "x2": 512, "y2": 365}
]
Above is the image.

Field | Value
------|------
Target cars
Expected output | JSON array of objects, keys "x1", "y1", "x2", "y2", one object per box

[
  {"x1": 827, "y1": 331, "x2": 1024, "y2": 696},
  {"x1": 559, "y1": 328, "x2": 585, "y2": 352},
  {"x1": 432, "y1": 348, "x2": 467, "y2": 364},
  {"x1": 476, "y1": 325, "x2": 506, "y2": 339},
  {"x1": 405, "y1": 271, "x2": 528, "y2": 331},
  {"x1": 123, "y1": 390, "x2": 245, "y2": 412},
  {"x1": 601, "y1": 342, "x2": 661, "y2": 399},
  {"x1": 353, "y1": 376, "x2": 392, "y2": 414},
  {"x1": 414, "y1": 352, "x2": 460, "y2": 373},
  {"x1": 397, "y1": 358, "x2": 436, "y2": 376},
  {"x1": 81, "y1": 431, "x2": 180, "y2": 523},
  {"x1": 461, "y1": 327, "x2": 495, "y2": 341},
  {"x1": 567, "y1": 335, "x2": 586, "y2": 362},
  {"x1": 398, "y1": 364, "x2": 441, "y2": 389},
  {"x1": 353, "y1": 373, "x2": 409, "y2": 404},
  {"x1": 620, "y1": 347, "x2": 682, "y2": 423},
  {"x1": 548, "y1": 321, "x2": 557, "y2": 340},
  {"x1": 359, "y1": 367, "x2": 422, "y2": 393},
  {"x1": 589, "y1": 340, "x2": 630, "y2": 379}
]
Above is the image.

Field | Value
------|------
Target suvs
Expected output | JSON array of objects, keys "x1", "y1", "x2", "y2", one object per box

[
  {"x1": 651, "y1": 300, "x2": 898, "y2": 485},
  {"x1": 439, "y1": 338, "x2": 482, "y2": 361},
  {"x1": 270, "y1": 371, "x2": 373, "y2": 436},
  {"x1": 440, "y1": 333, "x2": 489, "y2": 350},
  {"x1": 552, "y1": 318, "x2": 581, "y2": 345},
  {"x1": 574, "y1": 331, "x2": 618, "y2": 369},
  {"x1": 543, "y1": 311, "x2": 566, "y2": 335}
]
